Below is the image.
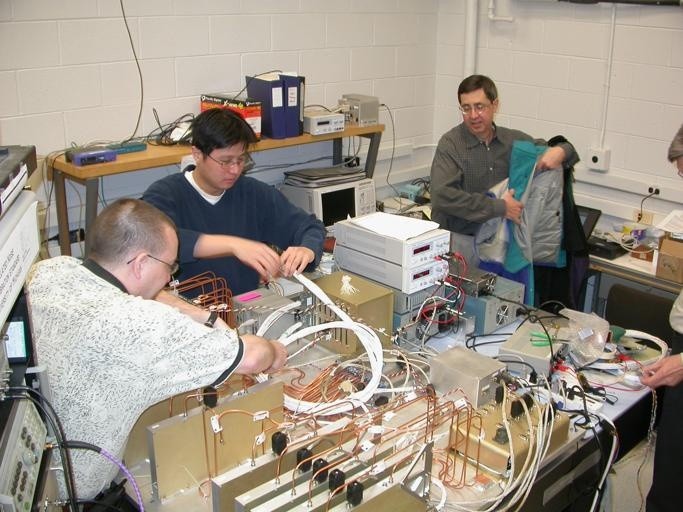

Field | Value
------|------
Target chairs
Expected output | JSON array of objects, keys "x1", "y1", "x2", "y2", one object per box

[{"x1": 540, "y1": 264, "x2": 601, "y2": 316}]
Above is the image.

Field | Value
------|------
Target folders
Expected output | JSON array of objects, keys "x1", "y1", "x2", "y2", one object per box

[
  {"x1": 277, "y1": 73, "x2": 300, "y2": 137},
  {"x1": 245, "y1": 73, "x2": 286, "y2": 139}
]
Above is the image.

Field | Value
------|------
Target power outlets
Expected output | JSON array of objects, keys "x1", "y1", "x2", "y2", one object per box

[{"x1": 634, "y1": 208, "x2": 653, "y2": 225}]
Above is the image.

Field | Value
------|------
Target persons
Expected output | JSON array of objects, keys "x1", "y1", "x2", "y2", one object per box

[
  {"x1": 27, "y1": 198, "x2": 288, "y2": 511},
  {"x1": 642, "y1": 126, "x2": 683, "y2": 510},
  {"x1": 144, "y1": 107, "x2": 328, "y2": 300},
  {"x1": 430, "y1": 72, "x2": 577, "y2": 238}
]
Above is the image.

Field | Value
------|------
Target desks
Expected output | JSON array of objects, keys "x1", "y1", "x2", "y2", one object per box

[{"x1": 115, "y1": 301, "x2": 670, "y2": 512}]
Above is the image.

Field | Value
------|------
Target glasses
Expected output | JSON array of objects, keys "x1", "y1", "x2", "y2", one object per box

[
  {"x1": 208, "y1": 152, "x2": 255, "y2": 170},
  {"x1": 126, "y1": 255, "x2": 179, "y2": 275},
  {"x1": 459, "y1": 105, "x2": 485, "y2": 114}
]
